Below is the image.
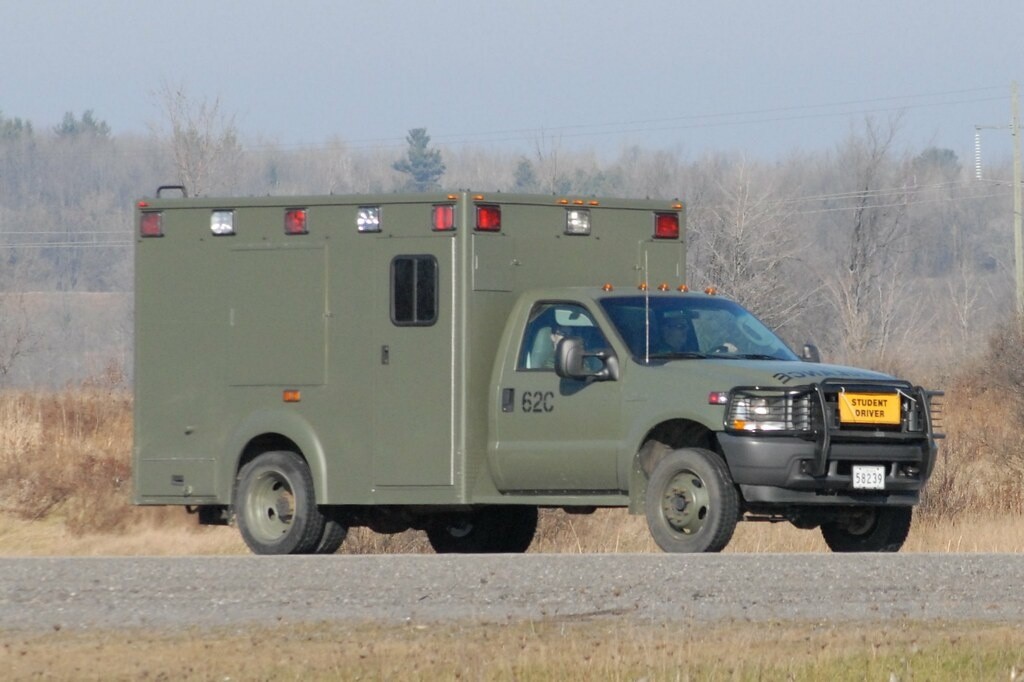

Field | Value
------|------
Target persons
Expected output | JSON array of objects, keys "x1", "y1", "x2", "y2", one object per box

[
  {"x1": 544, "y1": 322, "x2": 591, "y2": 370},
  {"x1": 648, "y1": 318, "x2": 737, "y2": 356}
]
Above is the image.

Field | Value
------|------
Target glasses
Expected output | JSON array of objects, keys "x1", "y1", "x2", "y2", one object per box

[
  {"x1": 659, "y1": 322, "x2": 687, "y2": 330},
  {"x1": 554, "y1": 330, "x2": 575, "y2": 337}
]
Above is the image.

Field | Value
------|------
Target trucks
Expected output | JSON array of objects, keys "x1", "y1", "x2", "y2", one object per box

[{"x1": 127, "y1": 182, "x2": 948, "y2": 556}]
